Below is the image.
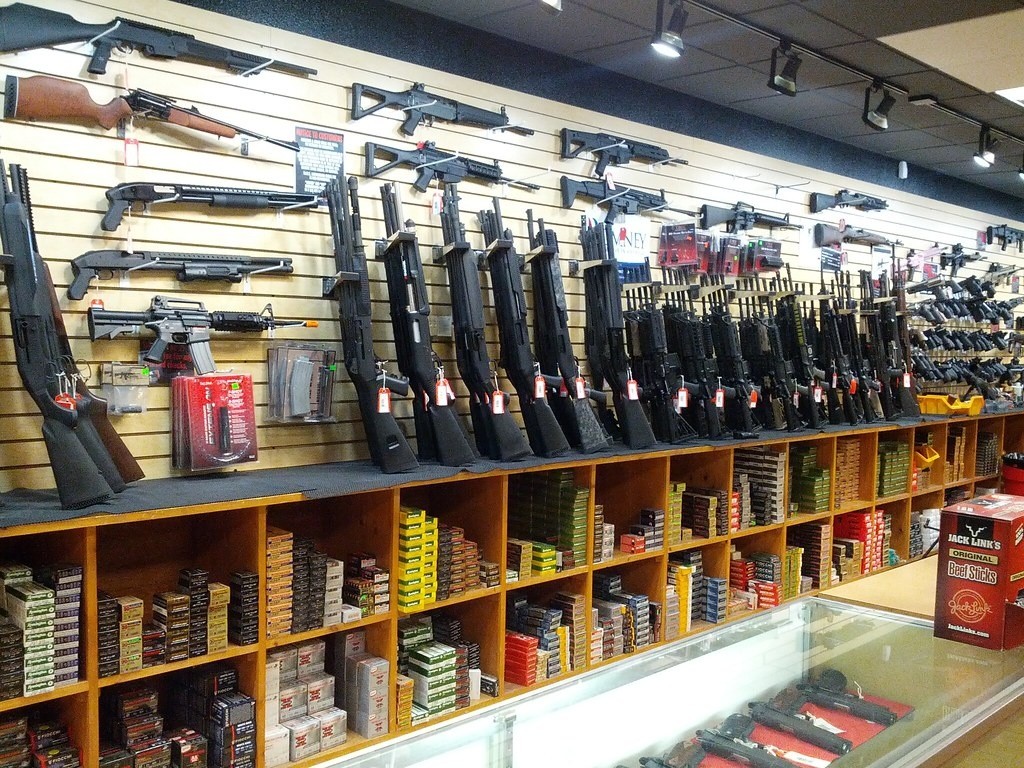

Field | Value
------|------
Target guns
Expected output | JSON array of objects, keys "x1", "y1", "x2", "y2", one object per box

[
  {"x1": 637, "y1": 667, "x2": 897, "y2": 767},
  {"x1": 1, "y1": 2, "x2": 1023, "y2": 505}
]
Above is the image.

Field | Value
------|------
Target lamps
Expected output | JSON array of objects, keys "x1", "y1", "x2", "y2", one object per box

[{"x1": 541, "y1": 0, "x2": 1024, "y2": 182}]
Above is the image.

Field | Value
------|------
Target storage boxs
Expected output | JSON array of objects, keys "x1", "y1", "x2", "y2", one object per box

[
  {"x1": 932, "y1": 493, "x2": 1024, "y2": 650},
  {"x1": 2, "y1": 425, "x2": 1003, "y2": 767}
]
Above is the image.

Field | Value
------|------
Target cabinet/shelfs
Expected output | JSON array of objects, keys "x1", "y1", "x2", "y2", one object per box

[{"x1": 1, "y1": 408, "x2": 1024, "y2": 768}]
